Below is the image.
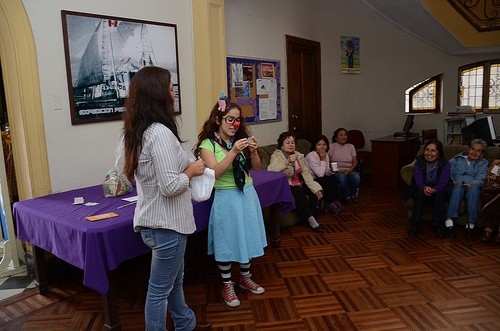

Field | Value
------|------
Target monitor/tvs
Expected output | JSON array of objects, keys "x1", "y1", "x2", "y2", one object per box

[
  {"x1": 403, "y1": 115, "x2": 416, "y2": 139},
  {"x1": 462, "y1": 116, "x2": 496, "y2": 145}
]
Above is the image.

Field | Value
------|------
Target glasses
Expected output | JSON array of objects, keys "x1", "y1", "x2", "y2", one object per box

[{"x1": 221, "y1": 115, "x2": 244, "y2": 124}]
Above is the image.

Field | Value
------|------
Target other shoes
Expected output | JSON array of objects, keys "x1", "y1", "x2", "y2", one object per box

[
  {"x1": 307, "y1": 202, "x2": 320, "y2": 216},
  {"x1": 194, "y1": 322, "x2": 210, "y2": 331},
  {"x1": 448, "y1": 231, "x2": 456, "y2": 237},
  {"x1": 465, "y1": 226, "x2": 475, "y2": 233},
  {"x1": 305, "y1": 217, "x2": 323, "y2": 232},
  {"x1": 352, "y1": 196, "x2": 359, "y2": 203},
  {"x1": 346, "y1": 199, "x2": 352, "y2": 205},
  {"x1": 328, "y1": 203, "x2": 342, "y2": 214},
  {"x1": 437, "y1": 227, "x2": 445, "y2": 238},
  {"x1": 409, "y1": 223, "x2": 418, "y2": 236},
  {"x1": 333, "y1": 200, "x2": 346, "y2": 210}
]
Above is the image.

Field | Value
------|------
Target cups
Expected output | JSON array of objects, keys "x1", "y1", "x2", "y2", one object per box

[{"x1": 331, "y1": 162, "x2": 337, "y2": 171}]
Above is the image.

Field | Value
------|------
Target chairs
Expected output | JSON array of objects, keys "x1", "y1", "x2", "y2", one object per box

[{"x1": 348, "y1": 130, "x2": 371, "y2": 180}]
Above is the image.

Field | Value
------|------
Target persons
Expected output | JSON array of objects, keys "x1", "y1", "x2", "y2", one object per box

[
  {"x1": 446, "y1": 139, "x2": 500, "y2": 242},
  {"x1": 408, "y1": 139, "x2": 450, "y2": 237},
  {"x1": 267, "y1": 132, "x2": 323, "y2": 232},
  {"x1": 195, "y1": 99, "x2": 268, "y2": 306},
  {"x1": 123, "y1": 66, "x2": 207, "y2": 331},
  {"x1": 305, "y1": 128, "x2": 360, "y2": 212}
]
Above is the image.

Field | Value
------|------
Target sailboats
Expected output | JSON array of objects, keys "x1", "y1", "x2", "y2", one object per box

[{"x1": 73, "y1": 17, "x2": 160, "y2": 118}]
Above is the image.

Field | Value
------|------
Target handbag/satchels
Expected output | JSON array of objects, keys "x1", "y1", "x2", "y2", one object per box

[
  {"x1": 189, "y1": 166, "x2": 215, "y2": 203},
  {"x1": 103, "y1": 154, "x2": 134, "y2": 198},
  {"x1": 485, "y1": 176, "x2": 500, "y2": 193}
]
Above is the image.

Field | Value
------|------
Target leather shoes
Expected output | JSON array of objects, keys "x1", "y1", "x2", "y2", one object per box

[
  {"x1": 494, "y1": 233, "x2": 500, "y2": 243},
  {"x1": 480, "y1": 231, "x2": 491, "y2": 242}
]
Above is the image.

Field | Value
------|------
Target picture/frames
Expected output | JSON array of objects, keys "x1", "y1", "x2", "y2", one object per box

[{"x1": 60, "y1": 9, "x2": 182, "y2": 126}]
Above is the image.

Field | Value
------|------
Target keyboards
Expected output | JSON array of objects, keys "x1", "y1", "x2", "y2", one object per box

[{"x1": 394, "y1": 132, "x2": 419, "y2": 136}]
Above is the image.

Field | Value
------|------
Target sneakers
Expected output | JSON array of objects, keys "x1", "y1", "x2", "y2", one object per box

[
  {"x1": 239, "y1": 274, "x2": 265, "y2": 294},
  {"x1": 222, "y1": 280, "x2": 241, "y2": 307}
]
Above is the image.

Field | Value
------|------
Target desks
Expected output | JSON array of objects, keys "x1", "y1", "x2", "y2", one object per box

[
  {"x1": 12, "y1": 172, "x2": 296, "y2": 331},
  {"x1": 369, "y1": 129, "x2": 437, "y2": 191}
]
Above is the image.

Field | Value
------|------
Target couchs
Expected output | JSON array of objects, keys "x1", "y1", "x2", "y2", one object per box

[
  {"x1": 258, "y1": 140, "x2": 324, "y2": 228},
  {"x1": 400, "y1": 144, "x2": 500, "y2": 231}
]
